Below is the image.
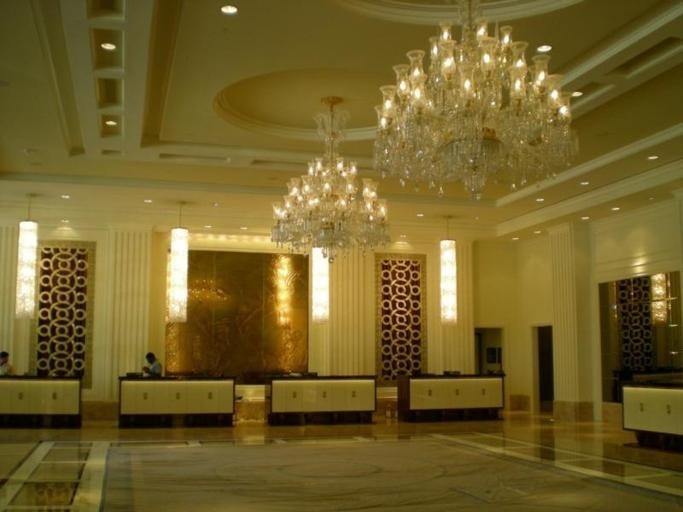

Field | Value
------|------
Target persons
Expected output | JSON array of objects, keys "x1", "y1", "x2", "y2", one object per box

[
  {"x1": 0, "y1": 351, "x2": 16, "y2": 376},
  {"x1": 142, "y1": 352, "x2": 162, "y2": 377}
]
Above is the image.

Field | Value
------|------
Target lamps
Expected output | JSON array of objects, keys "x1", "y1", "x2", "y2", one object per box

[
  {"x1": 168, "y1": 201, "x2": 188, "y2": 324},
  {"x1": 310, "y1": 247, "x2": 328, "y2": 321},
  {"x1": 14, "y1": 194, "x2": 37, "y2": 319},
  {"x1": 439, "y1": 216, "x2": 456, "y2": 323},
  {"x1": 270, "y1": 97, "x2": 390, "y2": 263},
  {"x1": 371, "y1": 1, "x2": 580, "y2": 199}
]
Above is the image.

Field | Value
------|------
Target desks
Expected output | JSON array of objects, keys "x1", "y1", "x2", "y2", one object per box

[
  {"x1": 397, "y1": 374, "x2": 503, "y2": 421},
  {"x1": 0, "y1": 376, "x2": 81, "y2": 429},
  {"x1": 118, "y1": 376, "x2": 234, "y2": 426},
  {"x1": 265, "y1": 375, "x2": 376, "y2": 424},
  {"x1": 622, "y1": 382, "x2": 682, "y2": 453}
]
